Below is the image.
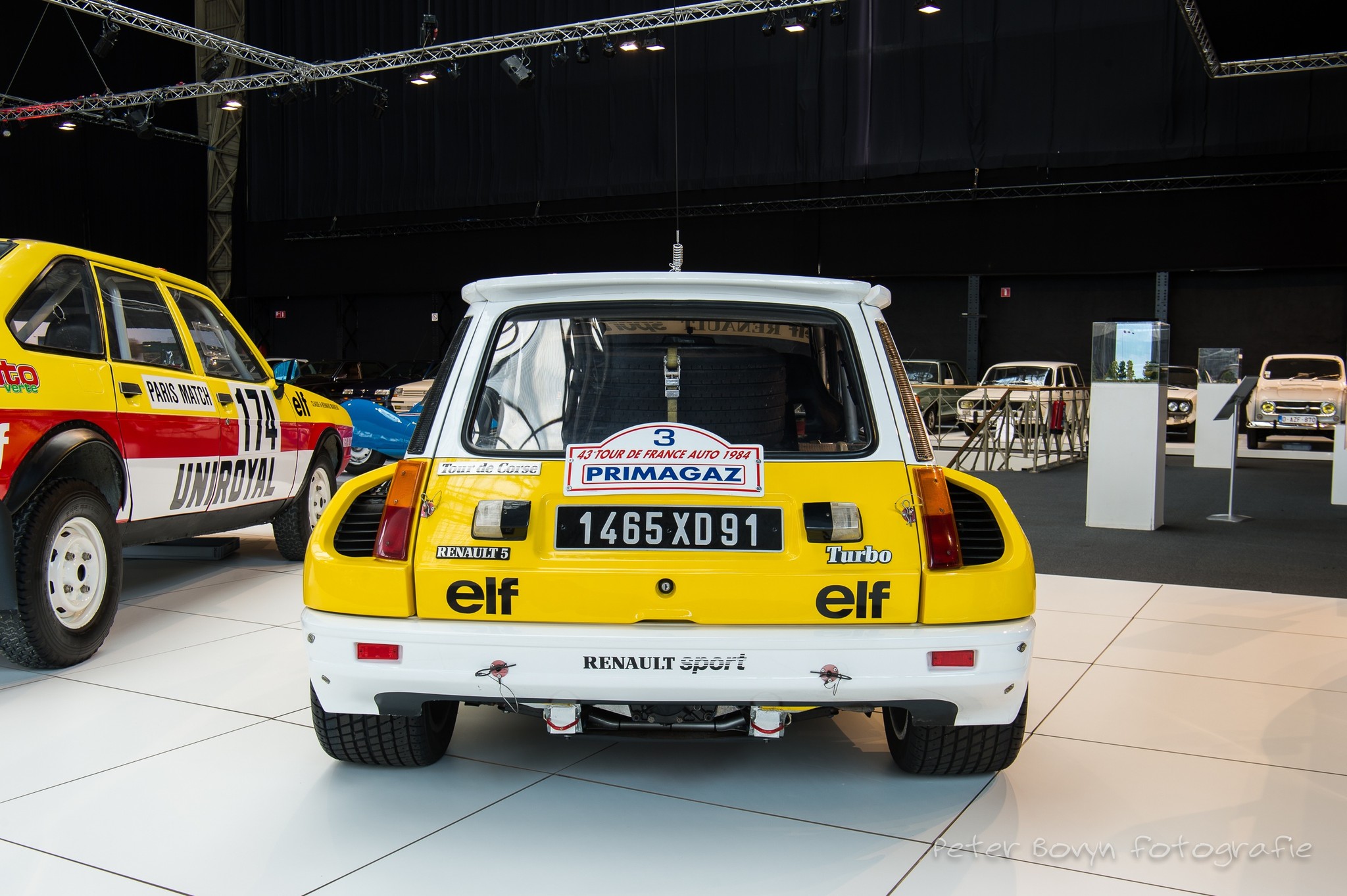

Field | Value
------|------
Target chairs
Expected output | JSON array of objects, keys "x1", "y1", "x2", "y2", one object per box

[{"x1": 43, "y1": 314, "x2": 121, "y2": 359}]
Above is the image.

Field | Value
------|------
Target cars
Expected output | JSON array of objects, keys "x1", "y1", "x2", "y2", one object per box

[
  {"x1": 955, "y1": 361, "x2": 1090, "y2": 441},
  {"x1": 1166, "y1": 366, "x2": 1199, "y2": 443},
  {"x1": 901, "y1": 359, "x2": 975, "y2": 434}
]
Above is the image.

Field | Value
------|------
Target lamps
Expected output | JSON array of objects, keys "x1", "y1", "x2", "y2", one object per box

[{"x1": 2, "y1": 0, "x2": 943, "y2": 137}]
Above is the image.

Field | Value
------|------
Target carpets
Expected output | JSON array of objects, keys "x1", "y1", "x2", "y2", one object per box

[{"x1": 962, "y1": 454, "x2": 1347, "y2": 599}]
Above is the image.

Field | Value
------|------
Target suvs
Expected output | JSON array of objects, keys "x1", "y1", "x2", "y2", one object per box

[
  {"x1": 1245, "y1": 354, "x2": 1347, "y2": 451},
  {"x1": 299, "y1": 270, "x2": 1032, "y2": 778},
  {"x1": 0, "y1": 238, "x2": 355, "y2": 671}
]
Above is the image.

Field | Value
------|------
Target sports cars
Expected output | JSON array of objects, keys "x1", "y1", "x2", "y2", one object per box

[{"x1": 273, "y1": 357, "x2": 499, "y2": 477}]
